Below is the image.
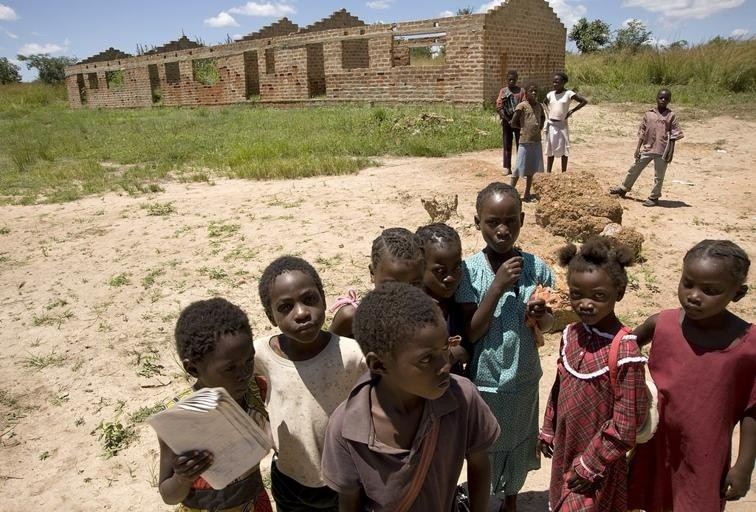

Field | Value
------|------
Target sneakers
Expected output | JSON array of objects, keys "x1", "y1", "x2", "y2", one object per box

[
  {"x1": 643, "y1": 198, "x2": 659, "y2": 207},
  {"x1": 610, "y1": 188, "x2": 626, "y2": 197}
]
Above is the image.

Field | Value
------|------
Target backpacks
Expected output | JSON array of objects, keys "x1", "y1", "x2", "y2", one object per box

[{"x1": 608, "y1": 328, "x2": 661, "y2": 443}]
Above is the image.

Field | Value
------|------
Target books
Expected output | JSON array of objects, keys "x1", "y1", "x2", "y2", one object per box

[{"x1": 146, "y1": 386, "x2": 273, "y2": 491}]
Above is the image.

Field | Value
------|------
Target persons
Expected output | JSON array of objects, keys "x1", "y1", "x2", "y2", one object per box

[
  {"x1": 495, "y1": 70, "x2": 588, "y2": 202},
  {"x1": 158, "y1": 298, "x2": 273, "y2": 511},
  {"x1": 454, "y1": 182, "x2": 555, "y2": 512},
  {"x1": 329, "y1": 222, "x2": 462, "y2": 339},
  {"x1": 319, "y1": 281, "x2": 503, "y2": 511},
  {"x1": 253, "y1": 255, "x2": 371, "y2": 511},
  {"x1": 609, "y1": 88, "x2": 685, "y2": 207},
  {"x1": 536, "y1": 238, "x2": 650, "y2": 511},
  {"x1": 631, "y1": 240, "x2": 755, "y2": 512}
]
